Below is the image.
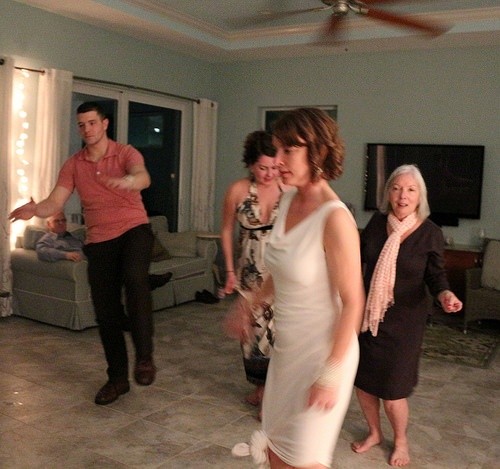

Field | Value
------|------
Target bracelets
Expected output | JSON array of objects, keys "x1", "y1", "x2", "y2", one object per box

[
  {"x1": 123, "y1": 175, "x2": 135, "y2": 189},
  {"x1": 315, "y1": 358, "x2": 344, "y2": 390},
  {"x1": 225, "y1": 270, "x2": 235, "y2": 273}
]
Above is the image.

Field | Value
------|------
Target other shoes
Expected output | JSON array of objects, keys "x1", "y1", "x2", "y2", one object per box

[
  {"x1": 195, "y1": 290, "x2": 215, "y2": 304},
  {"x1": 149, "y1": 271, "x2": 173, "y2": 292},
  {"x1": 201, "y1": 288, "x2": 220, "y2": 304},
  {"x1": 122, "y1": 315, "x2": 132, "y2": 333}
]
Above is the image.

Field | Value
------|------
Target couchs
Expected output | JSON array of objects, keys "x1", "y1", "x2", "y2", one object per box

[
  {"x1": 10, "y1": 215, "x2": 219, "y2": 331},
  {"x1": 426, "y1": 237, "x2": 500, "y2": 334}
]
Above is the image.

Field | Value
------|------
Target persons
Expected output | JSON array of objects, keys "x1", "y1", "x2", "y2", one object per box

[
  {"x1": 35, "y1": 212, "x2": 173, "y2": 332},
  {"x1": 222, "y1": 128, "x2": 292, "y2": 404},
  {"x1": 351, "y1": 165, "x2": 462, "y2": 468},
  {"x1": 223, "y1": 105, "x2": 365, "y2": 469},
  {"x1": 7, "y1": 99, "x2": 156, "y2": 405}
]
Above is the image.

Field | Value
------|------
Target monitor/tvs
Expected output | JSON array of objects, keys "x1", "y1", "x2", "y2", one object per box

[{"x1": 364, "y1": 143, "x2": 485, "y2": 227}]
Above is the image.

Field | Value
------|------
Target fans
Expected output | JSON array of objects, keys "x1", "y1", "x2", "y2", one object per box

[{"x1": 231, "y1": 0, "x2": 451, "y2": 46}]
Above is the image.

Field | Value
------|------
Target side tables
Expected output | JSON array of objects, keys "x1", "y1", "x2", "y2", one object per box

[{"x1": 196, "y1": 233, "x2": 223, "y2": 285}]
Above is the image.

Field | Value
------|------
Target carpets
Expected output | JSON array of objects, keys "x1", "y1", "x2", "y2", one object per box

[{"x1": 421, "y1": 300, "x2": 499, "y2": 369}]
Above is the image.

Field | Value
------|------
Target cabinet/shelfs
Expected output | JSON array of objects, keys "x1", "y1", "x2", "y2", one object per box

[{"x1": 445, "y1": 248, "x2": 483, "y2": 296}]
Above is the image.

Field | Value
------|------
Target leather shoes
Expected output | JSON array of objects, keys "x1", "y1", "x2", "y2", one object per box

[
  {"x1": 134, "y1": 357, "x2": 155, "y2": 385},
  {"x1": 95, "y1": 378, "x2": 131, "y2": 405}
]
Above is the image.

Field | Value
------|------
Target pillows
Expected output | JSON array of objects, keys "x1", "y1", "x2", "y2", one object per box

[
  {"x1": 480, "y1": 240, "x2": 500, "y2": 290},
  {"x1": 24, "y1": 226, "x2": 85, "y2": 250},
  {"x1": 156, "y1": 229, "x2": 197, "y2": 259}
]
